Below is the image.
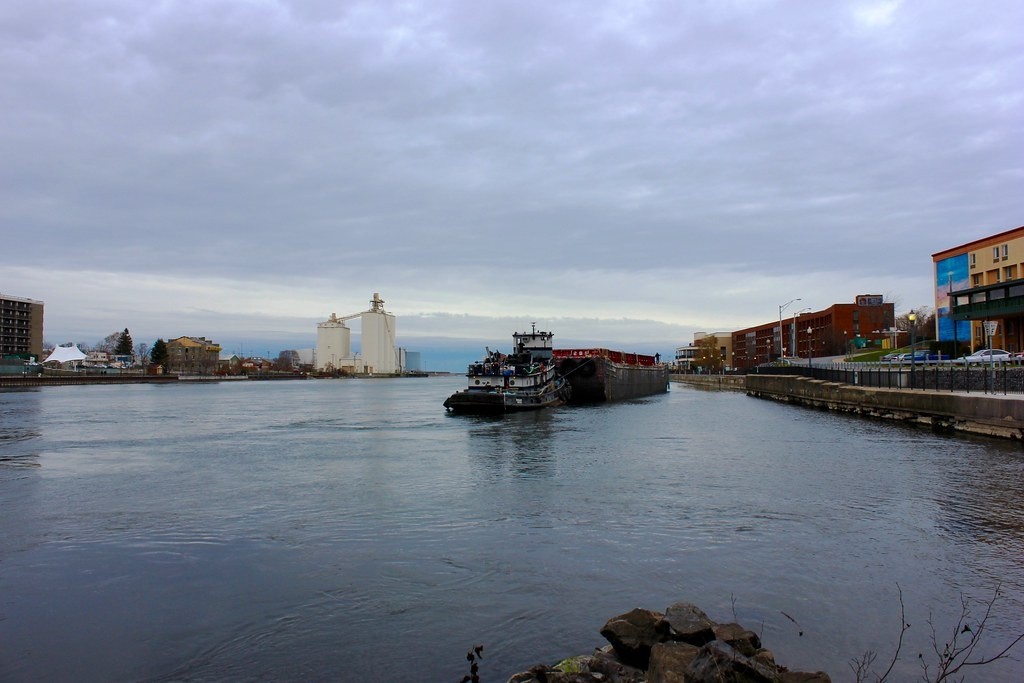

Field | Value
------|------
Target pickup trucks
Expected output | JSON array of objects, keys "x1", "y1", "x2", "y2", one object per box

[{"x1": 902, "y1": 350, "x2": 950, "y2": 366}]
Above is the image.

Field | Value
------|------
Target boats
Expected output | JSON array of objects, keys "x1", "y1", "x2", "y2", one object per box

[
  {"x1": 443, "y1": 322, "x2": 573, "y2": 413},
  {"x1": 553, "y1": 347, "x2": 670, "y2": 402}
]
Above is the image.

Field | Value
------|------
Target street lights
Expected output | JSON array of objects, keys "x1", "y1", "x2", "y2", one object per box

[
  {"x1": 732, "y1": 352, "x2": 735, "y2": 371},
  {"x1": 766, "y1": 338, "x2": 770, "y2": 363},
  {"x1": 982, "y1": 321, "x2": 999, "y2": 394},
  {"x1": 844, "y1": 330, "x2": 848, "y2": 354},
  {"x1": 745, "y1": 351, "x2": 748, "y2": 367},
  {"x1": 793, "y1": 308, "x2": 811, "y2": 357},
  {"x1": 909, "y1": 309, "x2": 916, "y2": 389},
  {"x1": 806, "y1": 327, "x2": 812, "y2": 367},
  {"x1": 778, "y1": 298, "x2": 802, "y2": 366},
  {"x1": 720, "y1": 354, "x2": 723, "y2": 375}
]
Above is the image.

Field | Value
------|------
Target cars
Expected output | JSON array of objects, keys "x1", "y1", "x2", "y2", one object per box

[
  {"x1": 1008, "y1": 351, "x2": 1024, "y2": 365},
  {"x1": 955, "y1": 349, "x2": 1011, "y2": 367},
  {"x1": 890, "y1": 353, "x2": 912, "y2": 363},
  {"x1": 882, "y1": 353, "x2": 900, "y2": 362}
]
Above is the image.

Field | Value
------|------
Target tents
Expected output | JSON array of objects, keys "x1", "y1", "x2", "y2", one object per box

[{"x1": 42, "y1": 342, "x2": 88, "y2": 371}]
